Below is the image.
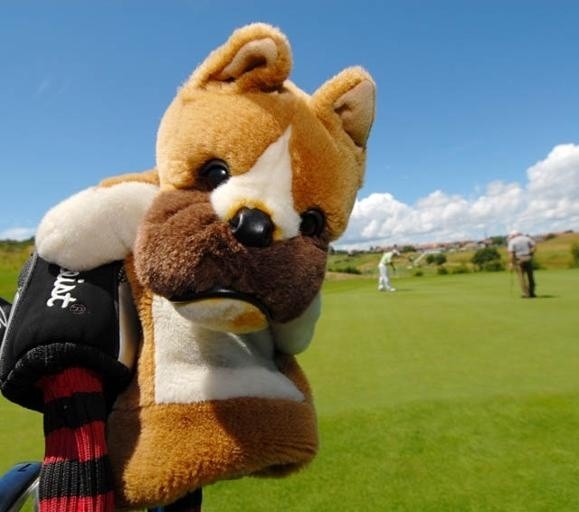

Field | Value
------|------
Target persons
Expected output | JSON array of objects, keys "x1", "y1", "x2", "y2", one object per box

[
  {"x1": 377, "y1": 250, "x2": 402, "y2": 291},
  {"x1": 507, "y1": 230, "x2": 538, "y2": 298}
]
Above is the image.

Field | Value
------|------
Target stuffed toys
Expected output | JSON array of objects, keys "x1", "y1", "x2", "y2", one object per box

[{"x1": 0, "y1": 23, "x2": 376, "y2": 511}]
